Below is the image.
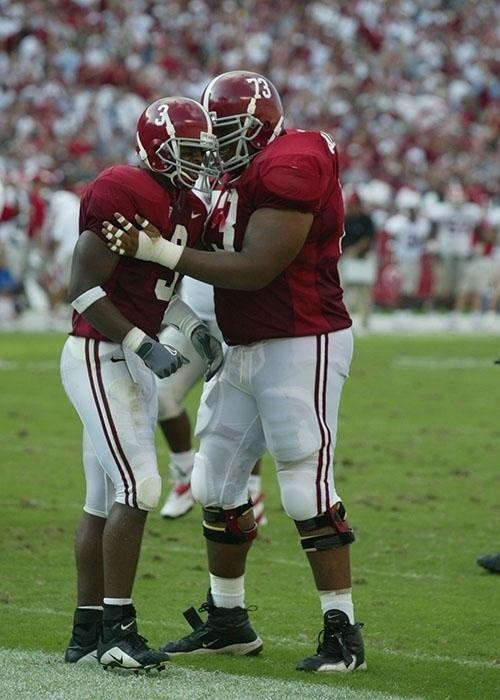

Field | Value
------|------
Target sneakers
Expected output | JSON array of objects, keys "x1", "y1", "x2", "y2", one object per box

[
  {"x1": 66, "y1": 636, "x2": 99, "y2": 663},
  {"x1": 159, "y1": 463, "x2": 195, "y2": 517},
  {"x1": 97, "y1": 617, "x2": 170, "y2": 673},
  {"x1": 295, "y1": 609, "x2": 367, "y2": 672},
  {"x1": 248, "y1": 494, "x2": 266, "y2": 526},
  {"x1": 160, "y1": 588, "x2": 263, "y2": 657}
]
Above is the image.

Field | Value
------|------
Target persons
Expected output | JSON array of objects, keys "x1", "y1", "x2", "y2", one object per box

[
  {"x1": 156, "y1": 275, "x2": 268, "y2": 526},
  {"x1": 103, "y1": 71, "x2": 366, "y2": 673},
  {"x1": 59, "y1": 95, "x2": 223, "y2": 672}
]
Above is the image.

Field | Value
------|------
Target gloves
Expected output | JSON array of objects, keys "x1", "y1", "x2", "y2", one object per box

[
  {"x1": 135, "y1": 335, "x2": 190, "y2": 379},
  {"x1": 190, "y1": 325, "x2": 222, "y2": 383}
]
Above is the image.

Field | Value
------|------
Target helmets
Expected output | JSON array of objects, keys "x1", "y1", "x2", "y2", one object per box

[
  {"x1": 200, "y1": 71, "x2": 285, "y2": 185},
  {"x1": 135, "y1": 97, "x2": 219, "y2": 194}
]
